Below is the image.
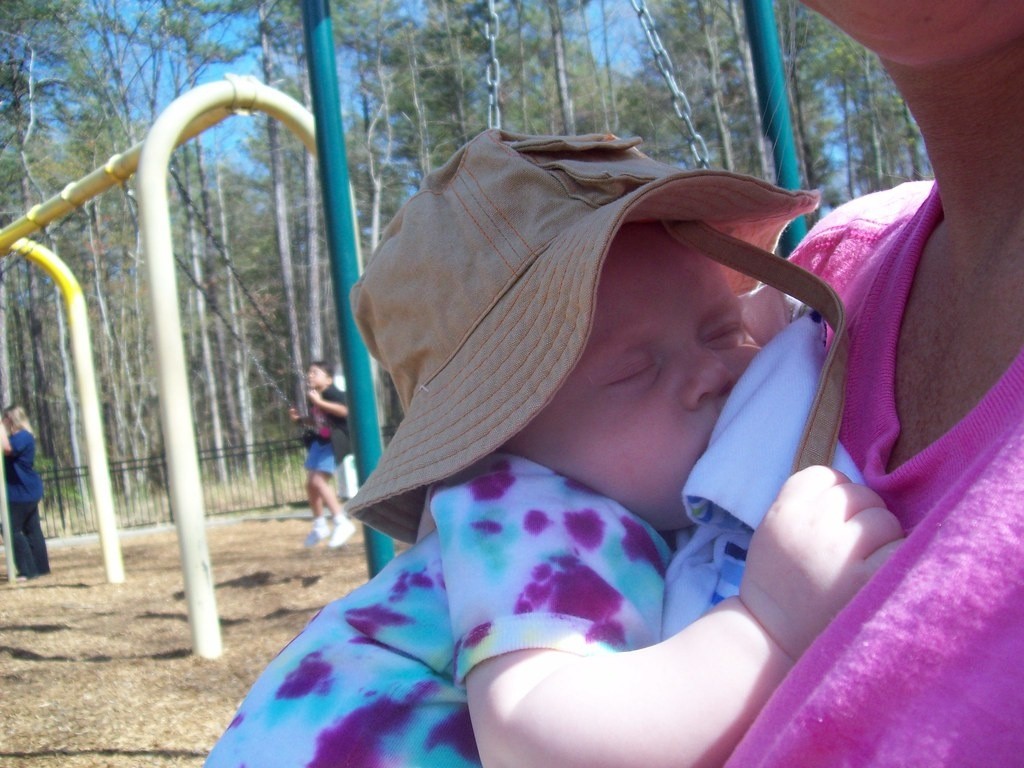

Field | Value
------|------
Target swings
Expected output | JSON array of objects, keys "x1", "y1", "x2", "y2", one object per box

[{"x1": 122, "y1": 158, "x2": 353, "y2": 462}]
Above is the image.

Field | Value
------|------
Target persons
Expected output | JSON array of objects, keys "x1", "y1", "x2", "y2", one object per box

[
  {"x1": 289, "y1": 359, "x2": 357, "y2": 552},
  {"x1": 709, "y1": 0, "x2": 1024, "y2": 767},
  {"x1": 0, "y1": 404, "x2": 52, "y2": 581},
  {"x1": 200, "y1": 129, "x2": 909, "y2": 768}
]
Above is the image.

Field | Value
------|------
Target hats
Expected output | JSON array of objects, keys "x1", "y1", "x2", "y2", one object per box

[{"x1": 345, "y1": 130, "x2": 850, "y2": 544}]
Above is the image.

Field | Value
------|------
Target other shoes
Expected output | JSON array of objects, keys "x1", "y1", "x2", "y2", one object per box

[
  {"x1": 15, "y1": 574, "x2": 37, "y2": 582},
  {"x1": 306, "y1": 525, "x2": 331, "y2": 545},
  {"x1": 329, "y1": 520, "x2": 356, "y2": 547}
]
